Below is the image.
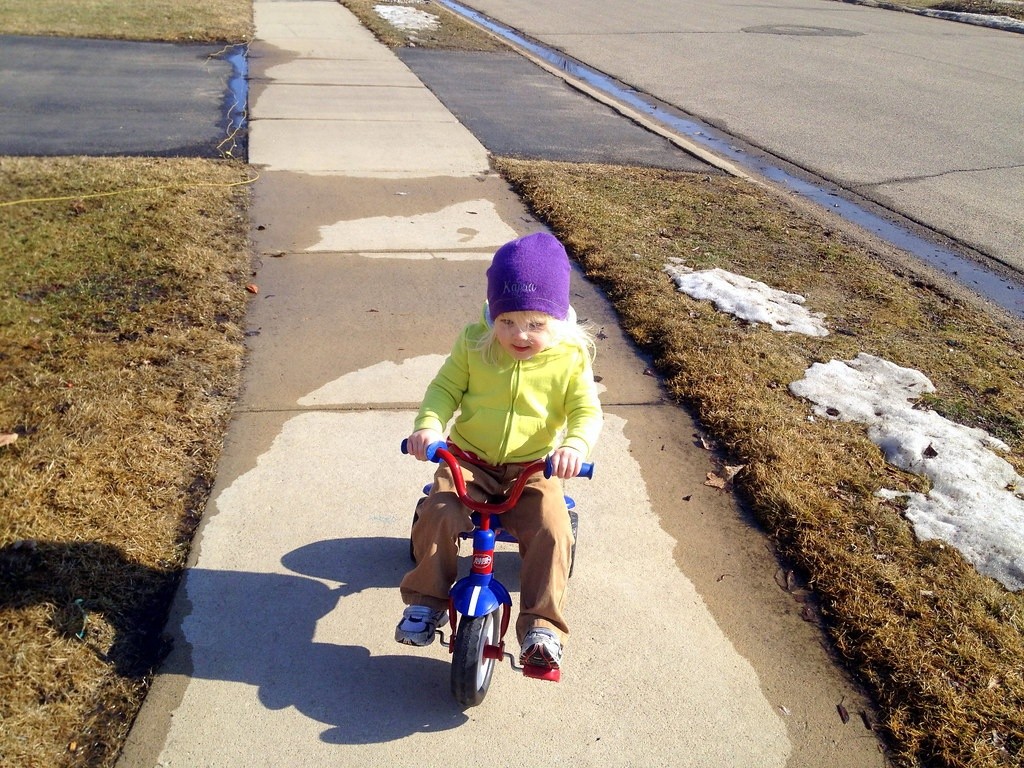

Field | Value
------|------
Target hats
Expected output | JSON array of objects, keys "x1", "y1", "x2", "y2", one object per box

[{"x1": 486, "y1": 232, "x2": 571, "y2": 322}]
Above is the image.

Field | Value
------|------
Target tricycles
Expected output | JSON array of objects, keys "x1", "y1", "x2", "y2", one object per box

[{"x1": 401, "y1": 438, "x2": 595, "y2": 707}]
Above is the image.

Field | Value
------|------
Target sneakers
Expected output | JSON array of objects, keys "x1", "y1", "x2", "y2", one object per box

[
  {"x1": 394, "y1": 605, "x2": 449, "y2": 647},
  {"x1": 519, "y1": 627, "x2": 563, "y2": 670}
]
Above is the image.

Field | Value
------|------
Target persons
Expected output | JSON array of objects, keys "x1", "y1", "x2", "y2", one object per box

[{"x1": 394, "y1": 232, "x2": 604, "y2": 682}]
